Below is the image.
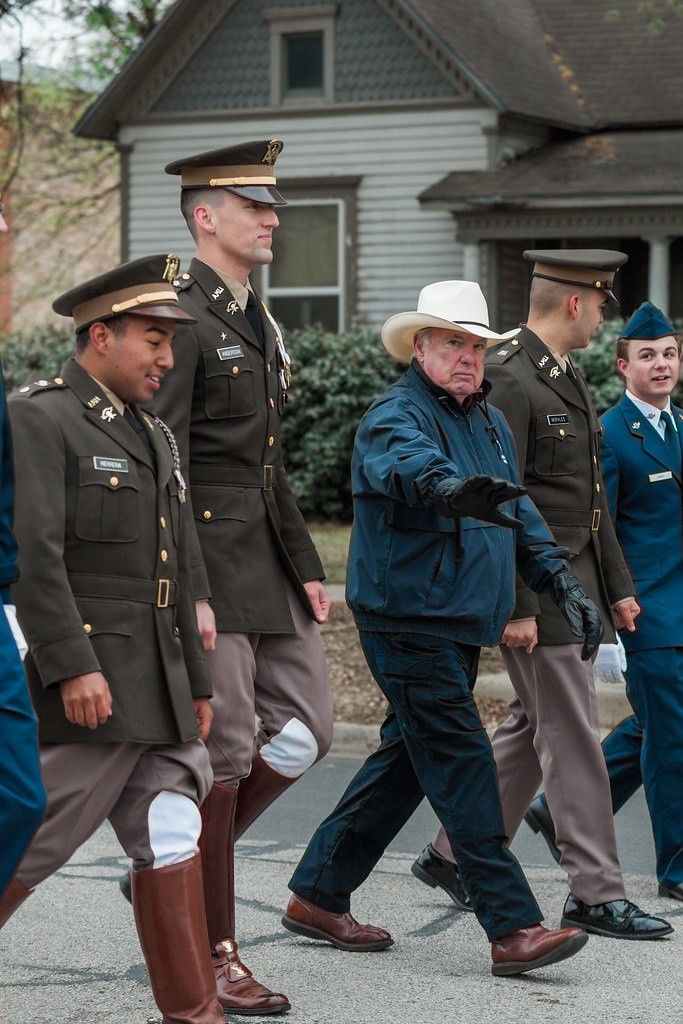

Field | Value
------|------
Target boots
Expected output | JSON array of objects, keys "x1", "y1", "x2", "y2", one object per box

[
  {"x1": 0, "y1": 877, "x2": 33, "y2": 929},
  {"x1": 200, "y1": 779, "x2": 296, "y2": 1016},
  {"x1": 118, "y1": 756, "x2": 282, "y2": 905},
  {"x1": 129, "y1": 852, "x2": 245, "y2": 1024}
]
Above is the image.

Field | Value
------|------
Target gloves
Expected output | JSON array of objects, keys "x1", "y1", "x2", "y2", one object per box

[
  {"x1": 428, "y1": 475, "x2": 525, "y2": 532},
  {"x1": 546, "y1": 572, "x2": 602, "y2": 662}
]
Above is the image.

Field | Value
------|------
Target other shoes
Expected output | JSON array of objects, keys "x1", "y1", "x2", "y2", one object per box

[{"x1": 523, "y1": 794, "x2": 568, "y2": 866}]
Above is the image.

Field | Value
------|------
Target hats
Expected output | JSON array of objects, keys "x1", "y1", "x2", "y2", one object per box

[
  {"x1": 526, "y1": 249, "x2": 625, "y2": 305},
  {"x1": 53, "y1": 254, "x2": 195, "y2": 331},
  {"x1": 167, "y1": 137, "x2": 289, "y2": 207},
  {"x1": 378, "y1": 278, "x2": 520, "y2": 368},
  {"x1": 619, "y1": 300, "x2": 675, "y2": 340}
]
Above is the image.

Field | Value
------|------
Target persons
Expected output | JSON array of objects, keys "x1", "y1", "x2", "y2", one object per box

[
  {"x1": 410, "y1": 249, "x2": 674, "y2": 939},
  {"x1": 141, "y1": 139, "x2": 333, "y2": 1013},
  {"x1": 521, "y1": 300, "x2": 683, "y2": 900},
  {"x1": 0, "y1": 253, "x2": 228, "y2": 1024},
  {"x1": 0, "y1": 211, "x2": 48, "y2": 899},
  {"x1": 282, "y1": 280, "x2": 590, "y2": 976}
]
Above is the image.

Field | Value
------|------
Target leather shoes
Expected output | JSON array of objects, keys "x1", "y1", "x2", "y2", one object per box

[
  {"x1": 281, "y1": 888, "x2": 394, "y2": 954},
  {"x1": 411, "y1": 844, "x2": 472, "y2": 912},
  {"x1": 488, "y1": 924, "x2": 586, "y2": 977},
  {"x1": 561, "y1": 893, "x2": 674, "y2": 942}
]
